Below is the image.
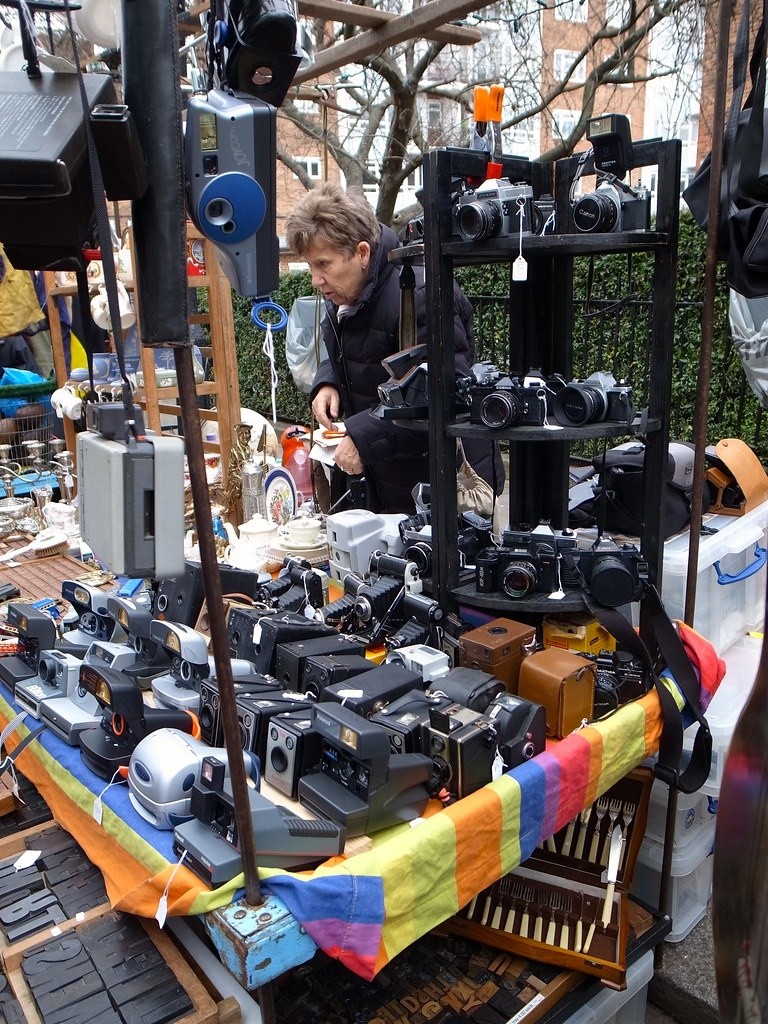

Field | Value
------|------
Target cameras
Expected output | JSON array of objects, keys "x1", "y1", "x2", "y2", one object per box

[
  {"x1": 0, "y1": 482, "x2": 648, "y2": 891},
  {"x1": 412, "y1": 114, "x2": 651, "y2": 241},
  {"x1": 377, "y1": 344, "x2": 634, "y2": 428}
]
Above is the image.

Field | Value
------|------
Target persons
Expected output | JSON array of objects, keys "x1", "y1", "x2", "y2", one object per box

[{"x1": 284, "y1": 183, "x2": 506, "y2": 515}]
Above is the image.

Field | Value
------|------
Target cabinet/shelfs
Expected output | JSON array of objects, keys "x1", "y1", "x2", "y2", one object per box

[
  {"x1": 387, "y1": 124, "x2": 678, "y2": 614},
  {"x1": 42, "y1": 221, "x2": 245, "y2": 541}
]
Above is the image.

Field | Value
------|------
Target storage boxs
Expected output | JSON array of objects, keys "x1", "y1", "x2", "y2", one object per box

[
  {"x1": 568, "y1": 473, "x2": 768, "y2": 946},
  {"x1": 558, "y1": 949, "x2": 657, "y2": 1023}
]
